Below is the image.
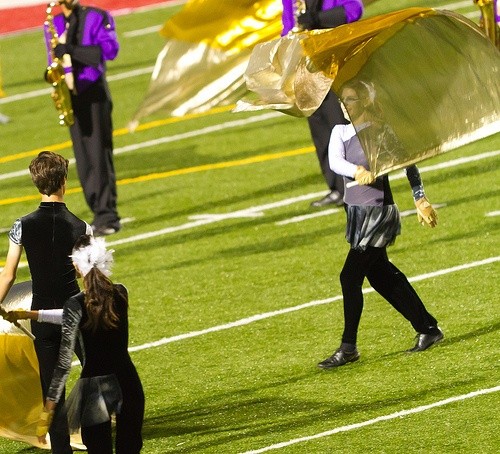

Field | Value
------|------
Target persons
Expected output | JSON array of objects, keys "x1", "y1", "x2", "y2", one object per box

[
  {"x1": 282, "y1": 0, "x2": 363, "y2": 207},
  {"x1": 4, "y1": 234, "x2": 145, "y2": 454},
  {"x1": 0, "y1": 151, "x2": 95, "y2": 454},
  {"x1": 318, "y1": 75, "x2": 444, "y2": 369},
  {"x1": 44, "y1": 0, "x2": 121, "y2": 235}
]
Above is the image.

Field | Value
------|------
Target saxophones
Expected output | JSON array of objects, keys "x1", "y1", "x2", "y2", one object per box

[{"x1": 42, "y1": 1, "x2": 78, "y2": 127}]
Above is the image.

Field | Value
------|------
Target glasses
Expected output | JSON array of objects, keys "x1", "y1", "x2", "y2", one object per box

[{"x1": 338, "y1": 97, "x2": 359, "y2": 103}]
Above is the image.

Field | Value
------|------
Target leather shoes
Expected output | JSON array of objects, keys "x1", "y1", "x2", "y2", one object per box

[
  {"x1": 317, "y1": 347, "x2": 360, "y2": 368},
  {"x1": 406, "y1": 327, "x2": 444, "y2": 352},
  {"x1": 310, "y1": 190, "x2": 345, "y2": 209}
]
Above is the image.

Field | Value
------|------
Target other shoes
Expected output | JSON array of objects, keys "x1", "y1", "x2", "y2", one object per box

[{"x1": 91, "y1": 223, "x2": 115, "y2": 236}]
